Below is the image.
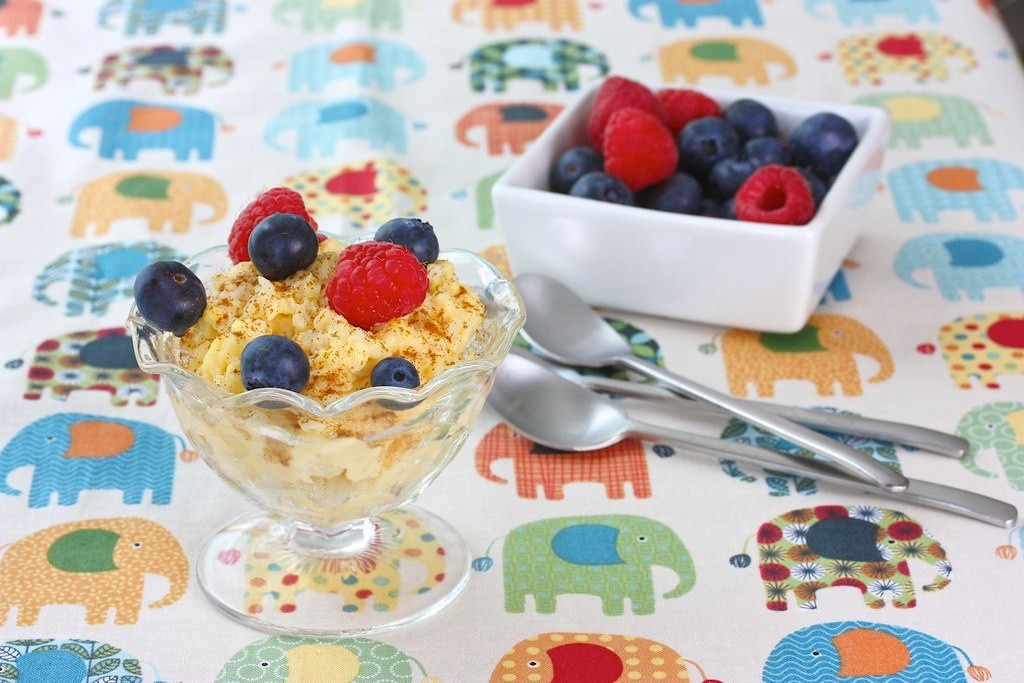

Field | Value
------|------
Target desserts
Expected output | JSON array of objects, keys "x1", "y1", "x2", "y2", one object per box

[{"x1": 127, "y1": 185, "x2": 490, "y2": 526}]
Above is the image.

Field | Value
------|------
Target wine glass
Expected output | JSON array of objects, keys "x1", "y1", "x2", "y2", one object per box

[{"x1": 124, "y1": 229, "x2": 526, "y2": 638}]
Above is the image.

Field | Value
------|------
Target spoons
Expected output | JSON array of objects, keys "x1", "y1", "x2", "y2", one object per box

[
  {"x1": 485, "y1": 354, "x2": 1018, "y2": 528},
  {"x1": 511, "y1": 272, "x2": 910, "y2": 493}
]
